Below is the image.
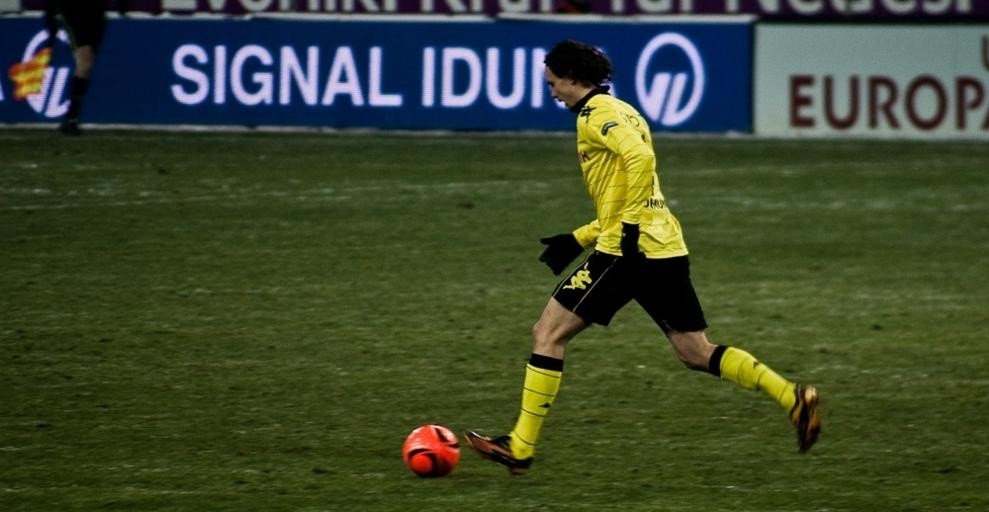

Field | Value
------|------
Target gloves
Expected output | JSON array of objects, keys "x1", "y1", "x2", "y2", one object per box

[
  {"x1": 539, "y1": 233, "x2": 583, "y2": 276},
  {"x1": 620, "y1": 221, "x2": 647, "y2": 266}
]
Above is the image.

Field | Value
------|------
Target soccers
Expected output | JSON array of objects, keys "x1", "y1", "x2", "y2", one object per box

[{"x1": 404, "y1": 424, "x2": 460, "y2": 477}]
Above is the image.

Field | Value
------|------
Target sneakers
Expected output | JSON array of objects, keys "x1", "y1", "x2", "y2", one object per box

[
  {"x1": 463, "y1": 430, "x2": 534, "y2": 475},
  {"x1": 789, "y1": 383, "x2": 822, "y2": 452}
]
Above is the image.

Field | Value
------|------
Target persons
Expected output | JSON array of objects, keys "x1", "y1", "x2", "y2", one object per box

[
  {"x1": 463, "y1": 38, "x2": 823, "y2": 478},
  {"x1": 39, "y1": 1, "x2": 110, "y2": 138}
]
